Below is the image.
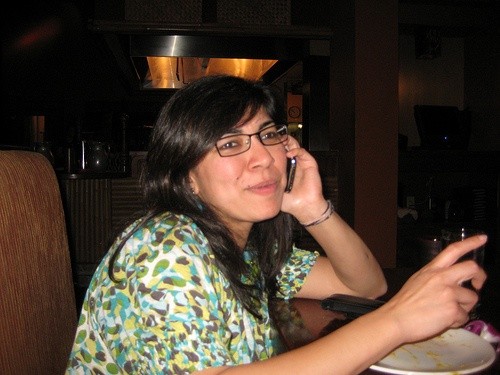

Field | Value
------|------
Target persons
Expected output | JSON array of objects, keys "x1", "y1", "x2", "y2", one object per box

[{"x1": 64, "y1": 74, "x2": 487, "y2": 375}]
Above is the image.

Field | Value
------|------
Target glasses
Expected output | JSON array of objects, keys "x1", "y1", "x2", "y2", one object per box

[{"x1": 212, "y1": 122, "x2": 289, "y2": 157}]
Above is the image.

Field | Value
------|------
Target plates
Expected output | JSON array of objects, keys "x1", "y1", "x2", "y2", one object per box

[{"x1": 368, "y1": 327, "x2": 497, "y2": 375}]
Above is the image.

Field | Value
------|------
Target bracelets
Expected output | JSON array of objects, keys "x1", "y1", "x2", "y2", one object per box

[{"x1": 298, "y1": 200, "x2": 334, "y2": 227}]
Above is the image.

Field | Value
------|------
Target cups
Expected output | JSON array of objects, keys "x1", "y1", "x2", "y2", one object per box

[{"x1": 440, "y1": 228, "x2": 485, "y2": 308}]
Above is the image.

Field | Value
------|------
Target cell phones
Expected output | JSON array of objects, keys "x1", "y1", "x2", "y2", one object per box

[{"x1": 285, "y1": 144, "x2": 297, "y2": 192}]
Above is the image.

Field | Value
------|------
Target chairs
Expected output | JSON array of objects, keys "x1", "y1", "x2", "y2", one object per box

[{"x1": 0, "y1": 151, "x2": 79, "y2": 375}]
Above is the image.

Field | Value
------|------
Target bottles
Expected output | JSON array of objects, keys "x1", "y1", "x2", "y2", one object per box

[{"x1": 25, "y1": 115, "x2": 55, "y2": 172}]
